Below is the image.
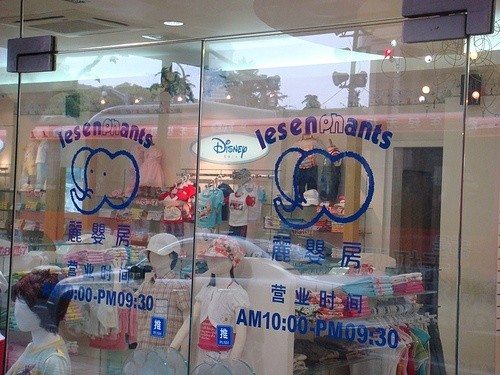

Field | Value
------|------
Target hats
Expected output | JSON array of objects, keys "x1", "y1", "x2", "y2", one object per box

[
  {"x1": 142, "y1": 233, "x2": 182, "y2": 256},
  {"x1": 197, "y1": 234, "x2": 246, "y2": 266}
]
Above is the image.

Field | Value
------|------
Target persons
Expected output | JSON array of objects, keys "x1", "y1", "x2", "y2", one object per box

[
  {"x1": 35, "y1": 137, "x2": 49, "y2": 190},
  {"x1": 130, "y1": 141, "x2": 165, "y2": 188},
  {"x1": 320, "y1": 146, "x2": 342, "y2": 206},
  {"x1": 124, "y1": 233, "x2": 197, "y2": 375},
  {"x1": 169, "y1": 235, "x2": 248, "y2": 375},
  {"x1": 291, "y1": 131, "x2": 319, "y2": 206},
  {"x1": 22, "y1": 137, "x2": 40, "y2": 190},
  {"x1": 2, "y1": 269, "x2": 75, "y2": 375}
]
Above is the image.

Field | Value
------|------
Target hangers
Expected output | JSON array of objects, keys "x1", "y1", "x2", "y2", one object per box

[
  {"x1": 161, "y1": 172, "x2": 266, "y2": 202},
  {"x1": 348, "y1": 302, "x2": 436, "y2": 336}
]
